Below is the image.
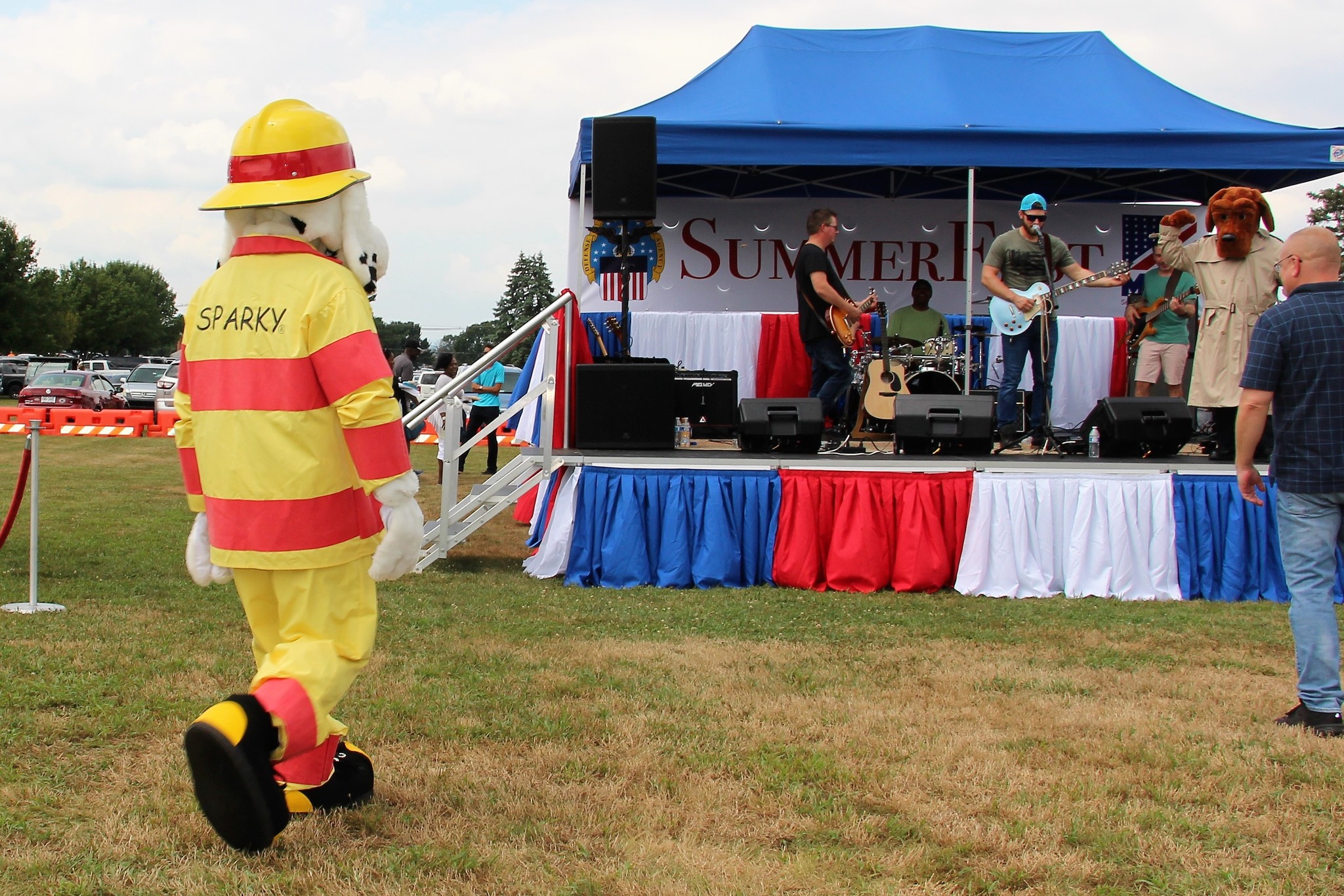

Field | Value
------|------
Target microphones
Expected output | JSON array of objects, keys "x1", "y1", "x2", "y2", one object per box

[
  {"x1": 700, "y1": 396, "x2": 705, "y2": 415},
  {"x1": 938, "y1": 320, "x2": 943, "y2": 336},
  {"x1": 1032, "y1": 225, "x2": 1044, "y2": 239}
]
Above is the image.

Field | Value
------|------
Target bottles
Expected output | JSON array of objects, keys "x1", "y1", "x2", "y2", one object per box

[
  {"x1": 680, "y1": 417, "x2": 691, "y2": 448},
  {"x1": 674, "y1": 417, "x2": 681, "y2": 448},
  {"x1": 1088, "y1": 427, "x2": 1099, "y2": 458}
]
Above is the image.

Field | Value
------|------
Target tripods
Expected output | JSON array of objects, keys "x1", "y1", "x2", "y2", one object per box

[{"x1": 994, "y1": 235, "x2": 1072, "y2": 458}]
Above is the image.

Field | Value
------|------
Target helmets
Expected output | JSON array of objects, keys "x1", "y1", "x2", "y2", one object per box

[{"x1": 199, "y1": 99, "x2": 372, "y2": 210}]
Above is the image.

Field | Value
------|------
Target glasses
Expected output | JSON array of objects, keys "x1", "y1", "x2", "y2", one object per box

[
  {"x1": 1023, "y1": 212, "x2": 1047, "y2": 222},
  {"x1": 483, "y1": 352, "x2": 489, "y2": 355},
  {"x1": 1273, "y1": 255, "x2": 1303, "y2": 273},
  {"x1": 827, "y1": 224, "x2": 838, "y2": 231}
]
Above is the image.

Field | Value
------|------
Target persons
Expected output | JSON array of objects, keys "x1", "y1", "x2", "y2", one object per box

[
  {"x1": 427, "y1": 352, "x2": 480, "y2": 486},
  {"x1": 883, "y1": 280, "x2": 952, "y2": 360},
  {"x1": 1234, "y1": 227, "x2": 1344, "y2": 736},
  {"x1": 982, "y1": 194, "x2": 1130, "y2": 451},
  {"x1": 796, "y1": 209, "x2": 878, "y2": 455},
  {"x1": 461, "y1": 344, "x2": 503, "y2": 475},
  {"x1": 7, "y1": 350, "x2": 15, "y2": 356},
  {"x1": 382, "y1": 338, "x2": 423, "y2": 476},
  {"x1": 78, "y1": 364, "x2": 86, "y2": 371},
  {"x1": 1125, "y1": 240, "x2": 1199, "y2": 396}
]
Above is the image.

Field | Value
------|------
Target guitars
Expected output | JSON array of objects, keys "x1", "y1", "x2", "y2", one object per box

[
  {"x1": 989, "y1": 259, "x2": 1134, "y2": 336},
  {"x1": 862, "y1": 301, "x2": 911, "y2": 421},
  {"x1": 1126, "y1": 283, "x2": 1200, "y2": 347},
  {"x1": 825, "y1": 286, "x2": 878, "y2": 347}
]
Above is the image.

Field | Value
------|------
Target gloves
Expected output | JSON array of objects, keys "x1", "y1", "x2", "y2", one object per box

[
  {"x1": 184, "y1": 511, "x2": 233, "y2": 587},
  {"x1": 367, "y1": 468, "x2": 423, "y2": 581}
]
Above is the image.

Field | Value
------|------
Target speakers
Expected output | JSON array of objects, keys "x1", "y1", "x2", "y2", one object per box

[
  {"x1": 575, "y1": 364, "x2": 676, "y2": 449},
  {"x1": 738, "y1": 397, "x2": 824, "y2": 453},
  {"x1": 675, "y1": 370, "x2": 739, "y2": 440},
  {"x1": 962, "y1": 389, "x2": 1026, "y2": 435},
  {"x1": 591, "y1": 116, "x2": 657, "y2": 221},
  {"x1": 1081, "y1": 397, "x2": 1193, "y2": 458},
  {"x1": 893, "y1": 394, "x2": 996, "y2": 455}
]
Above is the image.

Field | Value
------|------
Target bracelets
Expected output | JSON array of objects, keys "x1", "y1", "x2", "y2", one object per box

[
  {"x1": 1179, "y1": 312, "x2": 1189, "y2": 318},
  {"x1": 1125, "y1": 305, "x2": 1133, "y2": 309}
]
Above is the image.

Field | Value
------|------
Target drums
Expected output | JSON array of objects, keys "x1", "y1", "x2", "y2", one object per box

[
  {"x1": 906, "y1": 370, "x2": 962, "y2": 396},
  {"x1": 923, "y1": 336, "x2": 954, "y2": 366}
]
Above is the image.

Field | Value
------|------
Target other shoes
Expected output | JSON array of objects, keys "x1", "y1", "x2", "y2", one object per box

[
  {"x1": 185, "y1": 693, "x2": 293, "y2": 855},
  {"x1": 1262, "y1": 448, "x2": 1274, "y2": 463},
  {"x1": 1030, "y1": 438, "x2": 1054, "y2": 449},
  {"x1": 284, "y1": 740, "x2": 374, "y2": 813},
  {"x1": 1273, "y1": 698, "x2": 1344, "y2": 737},
  {"x1": 1208, "y1": 447, "x2": 1236, "y2": 460},
  {"x1": 1000, "y1": 437, "x2": 1022, "y2": 450},
  {"x1": 481, "y1": 468, "x2": 497, "y2": 474},
  {"x1": 412, "y1": 470, "x2": 425, "y2": 475}
]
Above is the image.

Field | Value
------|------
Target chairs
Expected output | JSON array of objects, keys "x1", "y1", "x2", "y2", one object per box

[
  {"x1": 72, "y1": 378, "x2": 82, "y2": 386},
  {"x1": 41, "y1": 378, "x2": 51, "y2": 387}
]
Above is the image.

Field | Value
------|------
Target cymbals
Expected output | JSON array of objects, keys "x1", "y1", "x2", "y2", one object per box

[
  {"x1": 952, "y1": 324, "x2": 987, "y2": 331},
  {"x1": 960, "y1": 332, "x2": 1001, "y2": 339},
  {"x1": 873, "y1": 336, "x2": 923, "y2": 348}
]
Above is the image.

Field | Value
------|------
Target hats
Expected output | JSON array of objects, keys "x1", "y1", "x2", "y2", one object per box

[{"x1": 1020, "y1": 193, "x2": 1048, "y2": 211}]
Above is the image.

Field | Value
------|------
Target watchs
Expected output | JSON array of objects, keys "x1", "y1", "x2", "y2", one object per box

[{"x1": 479, "y1": 385, "x2": 483, "y2": 390}]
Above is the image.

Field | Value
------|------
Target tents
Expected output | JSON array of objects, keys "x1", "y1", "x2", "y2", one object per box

[{"x1": 568, "y1": 28, "x2": 1344, "y2": 449}]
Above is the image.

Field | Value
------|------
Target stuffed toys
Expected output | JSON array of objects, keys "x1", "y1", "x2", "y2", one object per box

[
  {"x1": 1155, "y1": 186, "x2": 1290, "y2": 461},
  {"x1": 175, "y1": 101, "x2": 426, "y2": 858}
]
